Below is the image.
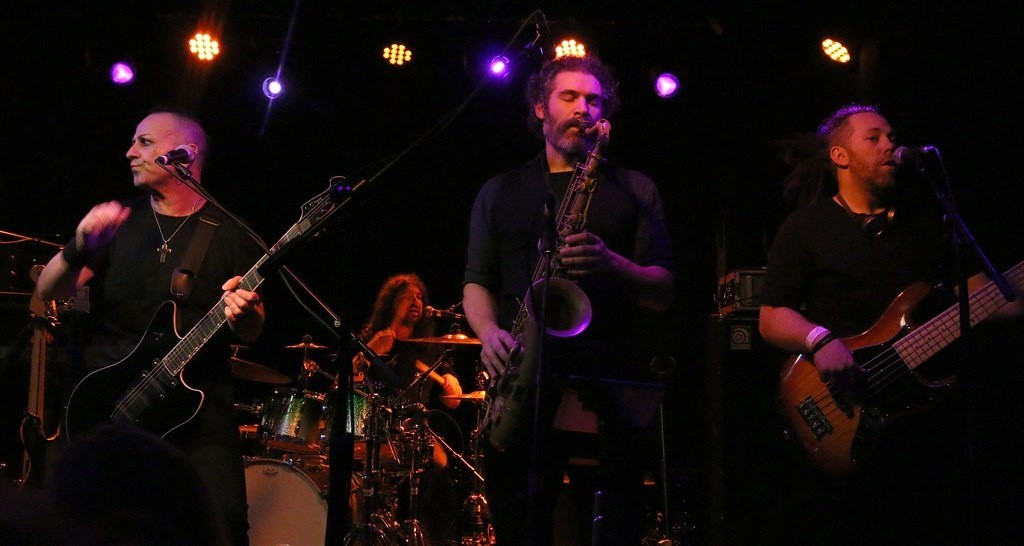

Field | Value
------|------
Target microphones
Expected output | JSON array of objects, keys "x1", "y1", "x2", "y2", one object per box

[
  {"x1": 426, "y1": 306, "x2": 466, "y2": 320},
  {"x1": 535, "y1": 11, "x2": 556, "y2": 61},
  {"x1": 156, "y1": 145, "x2": 195, "y2": 165},
  {"x1": 893, "y1": 146, "x2": 938, "y2": 164}
]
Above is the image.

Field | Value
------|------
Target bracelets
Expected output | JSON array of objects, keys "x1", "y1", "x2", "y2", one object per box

[
  {"x1": 806, "y1": 325, "x2": 837, "y2": 357},
  {"x1": 62, "y1": 243, "x2": 89, "y2": 264}
]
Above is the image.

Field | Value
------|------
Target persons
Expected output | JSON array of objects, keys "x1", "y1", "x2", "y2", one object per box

[
  {"x1": 461, "y1": 57, "x2": 676, "y2": 546},
  {"x1": 26, "y1": 112, "x2": 265, "y2": 545},
  {"x1": 759, "y1": 103, "x2": 1024, "y2": 545},
  {"x1": 347, "y1": 273, "x2": 468, "y2": 546}
]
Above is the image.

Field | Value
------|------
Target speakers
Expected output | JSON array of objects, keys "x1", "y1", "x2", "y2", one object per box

[{"x1": 706, "y1": 316, "x2": 797, "y2": 546}]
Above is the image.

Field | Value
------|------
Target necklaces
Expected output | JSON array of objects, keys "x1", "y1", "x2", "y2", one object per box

[
  {"x1": 837, "y1": 191, "x2": 897, "y2": 234},
  {"x1": 149, "y1": 193, "x2": 200, "y2": 262}
]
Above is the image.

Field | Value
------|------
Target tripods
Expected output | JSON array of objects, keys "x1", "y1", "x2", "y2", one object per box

[{"x1": 343, "y1": 336, "x2": 493, "y2": 546}]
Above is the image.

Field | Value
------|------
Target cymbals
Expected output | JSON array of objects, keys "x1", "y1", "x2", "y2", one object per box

[
  {"x1": 441, "y1": 391, "x2": 486, "y2": 405},
  {"x1": 398, "y1": 334, "x2": 481, "y2": 344},
  {"x1": 284, "y1": 343, "x2": 329, "y2": 349},
  {"x1": 218, "y1": 354, "x2": 293, "y2": 383}
]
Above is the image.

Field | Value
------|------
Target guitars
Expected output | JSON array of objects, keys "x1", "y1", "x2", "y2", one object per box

[
  {"x1": 778, "y1": 258, "x2": 1024, "y2": 472},
  {"x1": 65, "y1": 175, "x2": 360, "y2": 443}
]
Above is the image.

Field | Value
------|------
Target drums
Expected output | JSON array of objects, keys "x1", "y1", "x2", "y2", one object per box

[
  {"x1": 373, "y1": 408, "x2": 464, "y2": 471},
  {"x1": 243, "y1": 455, "x2": 367, "y2": 546},
  {"x1": 254, "y1": 385, "x2": 334, "y2": 454},
  {"x1": 344, "y1": 382, "x2": 402, "y2": 438},
  {"x1": 351, "y1": 466, "x2": 410, "y2": 524}
]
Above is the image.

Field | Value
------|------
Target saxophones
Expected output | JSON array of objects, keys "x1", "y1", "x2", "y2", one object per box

[{"x1": 474, "y1": 117, "x2": 613, "y2": 454}]
